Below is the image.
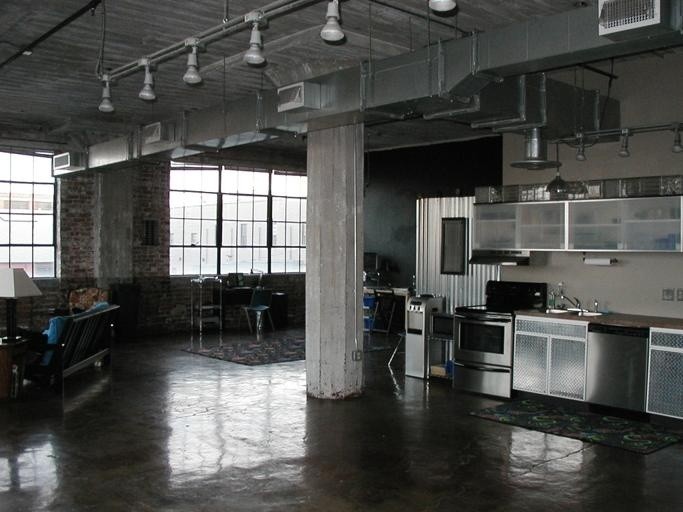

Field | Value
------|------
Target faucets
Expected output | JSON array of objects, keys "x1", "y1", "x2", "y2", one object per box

[{"x1": 561, "y1": 295, "x2": 580, "y2": 308}]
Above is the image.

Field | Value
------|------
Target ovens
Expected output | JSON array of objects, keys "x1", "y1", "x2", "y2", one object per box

[{"x1": 451, "y1": 320, "x2": 513, "y2": 399}]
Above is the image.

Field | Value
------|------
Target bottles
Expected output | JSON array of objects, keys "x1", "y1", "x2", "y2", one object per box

[{"x1": 548, "y1": 291, "x2": 554, "y2": 309}]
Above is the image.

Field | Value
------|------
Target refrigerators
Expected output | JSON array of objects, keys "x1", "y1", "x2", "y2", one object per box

[{"x1": 586, "y1": 322, "x2": 647, "y2": 417}]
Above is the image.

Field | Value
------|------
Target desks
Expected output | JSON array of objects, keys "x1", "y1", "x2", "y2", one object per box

[
  {"x1": 363, "y1": 288, "x2": 409, "y2": 331},
  {"x1": 214, "y1": 286, "x2": 253, "y2": 332},
  {"x1": 273, "y1": 293, "x2": 288, "y2": 328}
]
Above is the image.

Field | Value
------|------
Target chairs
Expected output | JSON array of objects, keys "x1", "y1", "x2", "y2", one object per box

[
  {"x1": 238, "y1": 288, "x2": 275, "y2": 335},
  {"x1": 47, "y1": 288, "x2": 118, "y2": 320},
  {"x1": 370, "y1": 288, "x2": 403, "y2": 337}
]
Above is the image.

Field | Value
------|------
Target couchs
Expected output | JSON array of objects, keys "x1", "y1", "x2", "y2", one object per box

[{"x1": 24, "y1": 302, "x2": 120, "y2": 398}]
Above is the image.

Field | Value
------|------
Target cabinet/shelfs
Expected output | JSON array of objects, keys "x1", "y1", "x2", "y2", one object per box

[
  {"x1": 190, "y1": 276, "x2": 222, "y2": 332},
  {"x1": 474, "y1": 197, "x2": 683, "y2": 252},
  {"x1": 427, "y1": 334, "x2": 455, "y2": 387}
]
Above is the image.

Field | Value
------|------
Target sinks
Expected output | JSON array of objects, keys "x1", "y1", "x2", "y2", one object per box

[
  {"x1": 570, "y1": 311, "x2": 609, "y2": 318},
  {"x1": 531, "y1": 308, "x2": 568, "y2": 314}
]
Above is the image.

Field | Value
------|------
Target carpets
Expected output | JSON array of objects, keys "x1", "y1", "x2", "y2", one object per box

[
  {"x1": 468, "y1": 393, "x2": 683, "y2": 454},
  {"x1": 182, "y1": 328, "x2": 404, "y2": 365}
]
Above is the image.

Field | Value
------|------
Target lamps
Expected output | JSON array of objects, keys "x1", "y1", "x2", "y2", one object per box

[
  {"x1": 0, "y1": 267, "x2": 43, "y2": 342},
  {"x1": 575, "y1": 121, "x2": 683, "y2": 160},
  {"x1": 95, "y1": 1, "x2": 460, "y2": 112}
]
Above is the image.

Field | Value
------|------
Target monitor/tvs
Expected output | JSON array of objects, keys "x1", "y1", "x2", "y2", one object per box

[{"x1": 364, "y1": 252, "x2": 378, "y2": 277}]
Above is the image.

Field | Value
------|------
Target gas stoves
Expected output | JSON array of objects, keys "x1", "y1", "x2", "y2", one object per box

[{"x1": 455, "y1": 304, "x2": 537, "y2": 319}]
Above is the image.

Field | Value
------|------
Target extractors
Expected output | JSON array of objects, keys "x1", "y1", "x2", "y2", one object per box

[{"x1": 468, "y1": 251, "x2": 546, "y2": 267}]
